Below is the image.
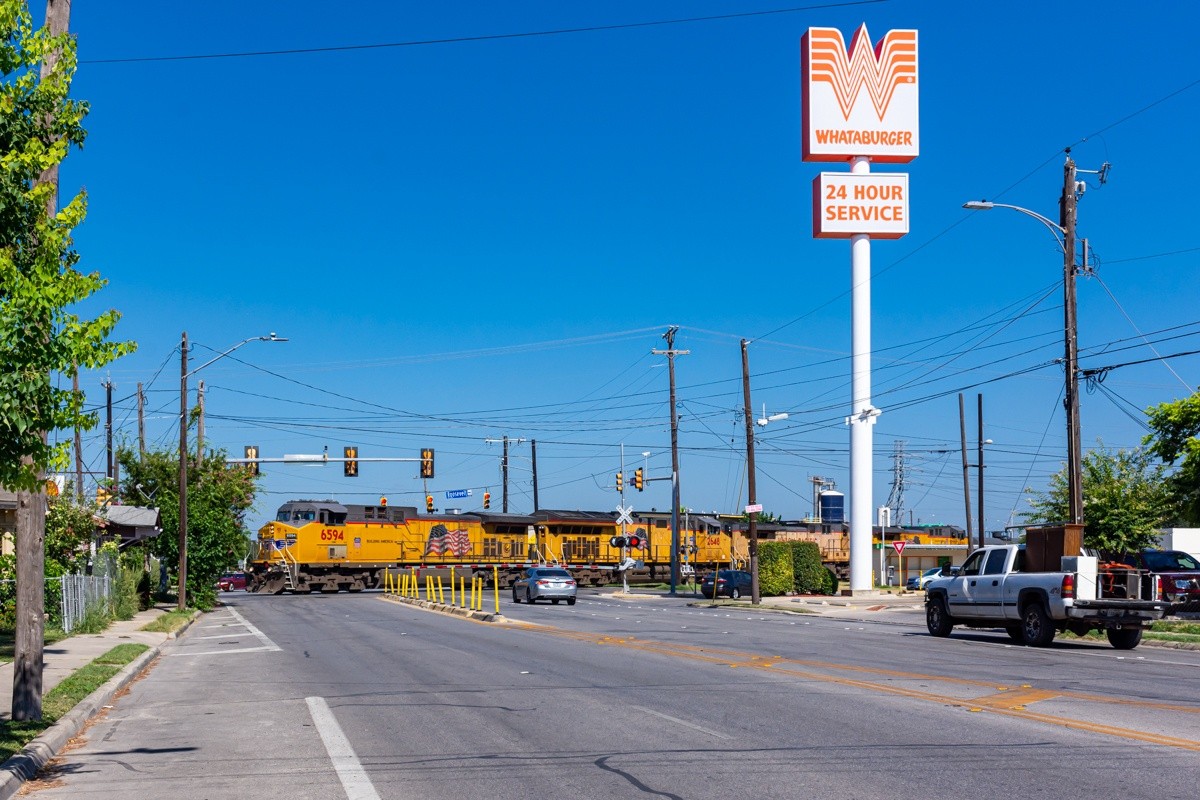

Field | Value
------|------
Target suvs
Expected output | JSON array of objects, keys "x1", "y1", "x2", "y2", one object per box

[{"x1": 700, "y1": 570, "x2": 753, "y2": 600}]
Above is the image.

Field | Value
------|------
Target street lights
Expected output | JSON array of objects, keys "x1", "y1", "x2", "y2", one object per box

[
  {"x1": 977, "y1": 438, "x2": 993, "y2": 549},
  {"x1": 963, "y1": 199, "x2": 1084, "y2": 528},
  {"x1": 178, "y1": 332, "x2": 289, "y2": 610},
  {"x1": 670, "y1": 413, "x2": 686, "y2": 587}
]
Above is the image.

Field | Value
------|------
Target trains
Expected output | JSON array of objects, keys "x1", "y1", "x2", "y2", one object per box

[{"x1": 252, "y1": 499, "x2": 976, "y2": 596}]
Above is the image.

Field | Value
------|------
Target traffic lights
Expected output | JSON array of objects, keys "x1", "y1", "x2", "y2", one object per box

[
  {"x1": 630, "y1": 537, "x2": 640, "y2": 547},
  {"x1": 615, "y1": 537, "x2": 627, "y2": 548},
  {"x1": 380, "y1": 497, "x2": 387, "y2": 508},
  {"x1": 97, "y1": 487, "x2": 114, "y2": 507},
  {"x1": 427, "y1": 495, "x2": 434, "y2": 511},
  {"x1": 616, "y1": 473, "x2": 622, "y2": 493},
  {"x1": 635, "y1": 467, "x2": 643, "y2": 491},
  {"x1": 484, "y1": 492, "x2": 491, "y2": 509}
]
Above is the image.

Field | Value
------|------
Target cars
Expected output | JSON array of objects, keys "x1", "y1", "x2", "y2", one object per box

[
  {"x1": 907, "y1": 566, "x2": 963, "y2": 591},
  {"x1": 1135, "y1": 549, "x2": 1200, "y2": 614},
  {"x1": 216, "y1": 574, "x2": 248, "y2": 592},
  {"x1": 512, "y1": 568, "x2": 578, "y2": 605}
]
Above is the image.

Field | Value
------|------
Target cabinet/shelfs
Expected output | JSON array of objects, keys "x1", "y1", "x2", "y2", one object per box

[{"x1": 1025, "y1": 524, "x2": 1085, "y2": 573}]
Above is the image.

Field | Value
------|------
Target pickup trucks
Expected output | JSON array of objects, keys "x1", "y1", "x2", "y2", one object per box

[{"x1": 923, "y1": 543, "x2": 1164, "y2": 649}]
